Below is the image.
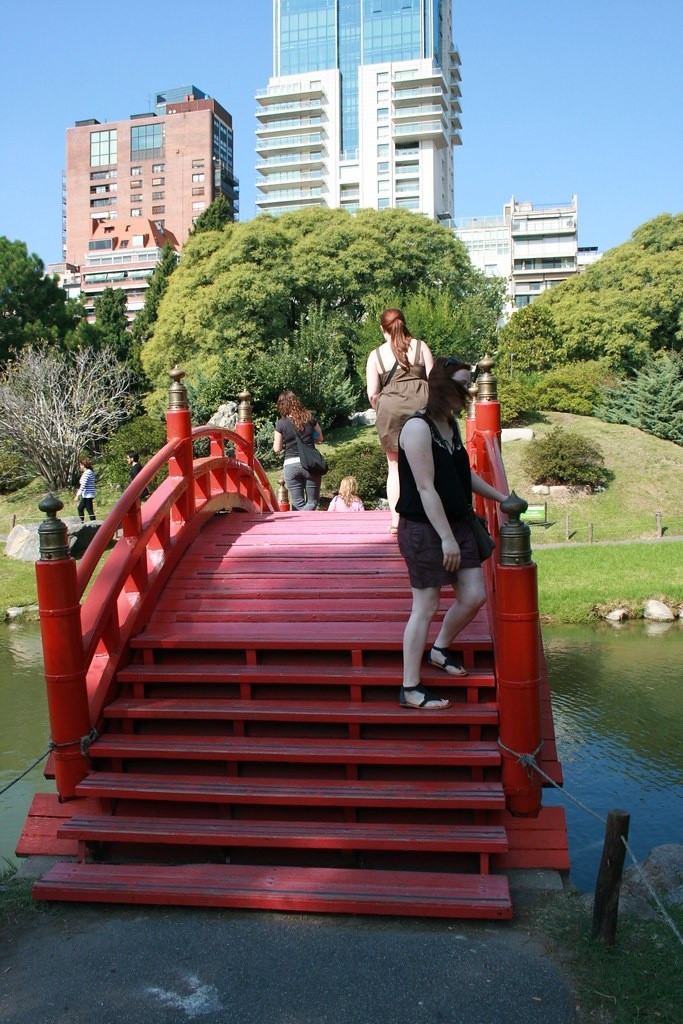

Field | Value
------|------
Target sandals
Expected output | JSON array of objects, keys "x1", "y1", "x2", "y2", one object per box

[
  {"x1": 399, "y1": 682, "x2": 451, "y2": 710},
  {"x1": 390, "y1": 526, "x2": 398, "y2": 536},
  {"x1": 427, "y1": 646, "x2": 469, "y2": 676}
]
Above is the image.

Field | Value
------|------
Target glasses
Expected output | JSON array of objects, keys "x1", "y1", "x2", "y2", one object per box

[{"x1": 444, "y1": 357, "x2": 465, "y2": 368}]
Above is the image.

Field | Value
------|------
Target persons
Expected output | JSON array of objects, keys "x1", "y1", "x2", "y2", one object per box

[
  {"x1": 394, "y1": 356, "x2": 511, "y2": 708},
  {"x1": 74, "y1": 459, "x2": 96, "y2": 523},
  {"x1": 327, "y1": 476, "x2": 364, "y2": 511},
  {"x1": 366, "y1": 308, "x2": 435, "y2": 537},
  {"x1": 126, "y1": 450, "x2": 149, "y2": 505},
  {"x1": 273, "y1": 391, "x2": 324, "y2": 511}
]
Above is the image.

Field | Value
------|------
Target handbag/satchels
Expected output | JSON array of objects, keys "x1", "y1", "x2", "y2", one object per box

[
  {"x1": 295, "y1": 435, "x2": 329, "y2": 476},
  {"x1": 139, "y1": 487, "x2": 149, "y2": 497},
  {"x1": 471, "y1": 513, "x2": 497, "y2": 563}
]
